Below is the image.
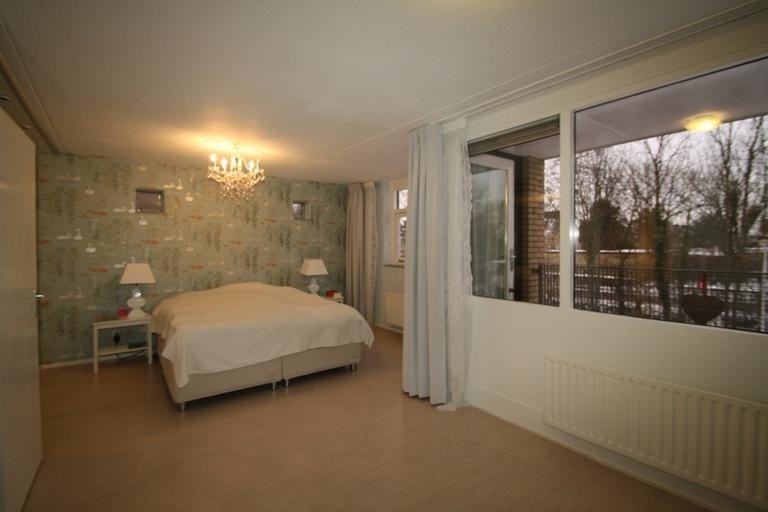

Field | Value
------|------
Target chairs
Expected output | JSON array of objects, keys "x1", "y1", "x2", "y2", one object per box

[{"x1": 613, "y1": 280, "x2": 651, "y2": 318}]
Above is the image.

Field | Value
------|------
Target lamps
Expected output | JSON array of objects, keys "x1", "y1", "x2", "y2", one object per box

[
  {"x1": 206, "y1": 142, "x2": 266, "y2": 207},
  {"x1": 301, "y1": 259, "x2": 330, "y2": 297},
  {"x1": 120, "y1": 263, "x2": 156, "y2": 322},
  {"x1": 680, "y1": 111, "x2": 724, "y2": 135}
]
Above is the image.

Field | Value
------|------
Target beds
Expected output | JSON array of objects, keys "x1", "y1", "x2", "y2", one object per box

[
  {"x1": 151, "y1": 282, "x2": 282, "y2": 412},
  {"x1": 228, "y1": 282, "x2": 375, "y2": 386}
]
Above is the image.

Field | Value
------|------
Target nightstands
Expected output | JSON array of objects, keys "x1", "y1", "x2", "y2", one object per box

[
  {"x1": 92, "y1": 313, "x2": 153, "y2": 375},
  {"x1": 326, "y1": 296, "x2": 344, "y2": 304}
]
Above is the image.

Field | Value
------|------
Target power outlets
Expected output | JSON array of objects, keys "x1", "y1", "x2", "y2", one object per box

[{"x1": 111, "y1": 329, "x2": 121, "y2": 344}]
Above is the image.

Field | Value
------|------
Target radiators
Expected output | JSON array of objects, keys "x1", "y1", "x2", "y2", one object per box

[{"x1": 543, "y1": 356, "x2": 768, "y2": 512}]
